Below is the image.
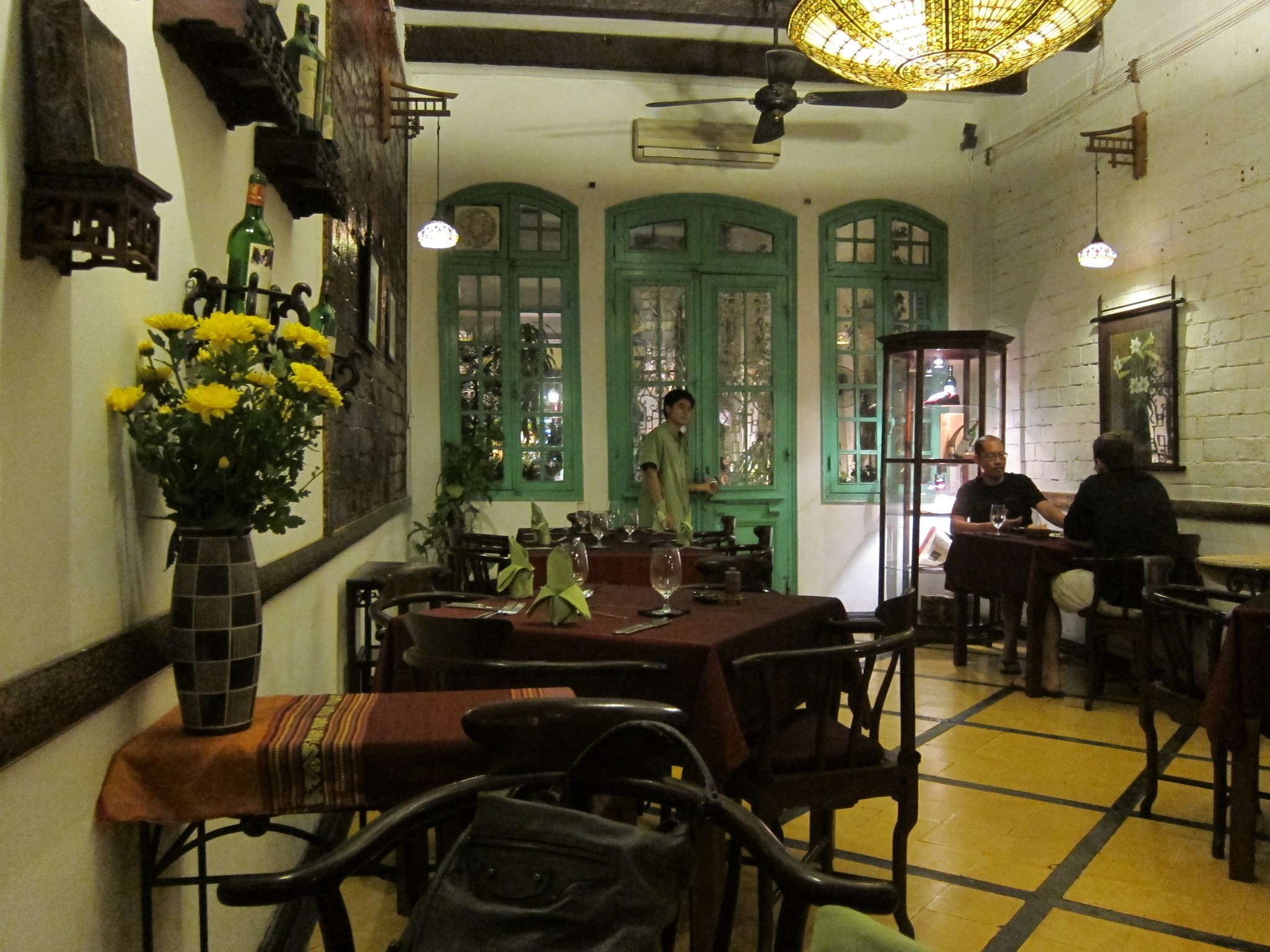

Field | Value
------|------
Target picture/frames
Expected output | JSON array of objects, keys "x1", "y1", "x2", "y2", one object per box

[{"x1": 1090, "y1": 274, "x2": 1186, "y2": 470}]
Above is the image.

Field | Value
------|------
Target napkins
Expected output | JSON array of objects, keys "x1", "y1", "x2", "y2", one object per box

[
  {"x1": 652, "y1": 499, "x2": 667, "y2": 533},
  {"x1": 530, "y1": 500, "x2": 551, "y2": 546},
  {"x1": 676, "y1": 504, "x2": 695, "y2": 546},
  {"x1": 526, "y1": 544, "x2": 591, "y2": 625},
  {"x1": 495, "y1": 534, "x2": 535, "y2": 598}
]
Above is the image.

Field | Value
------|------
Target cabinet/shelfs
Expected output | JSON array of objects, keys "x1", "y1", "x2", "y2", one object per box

[
  {"x1": 876, "y1": 329, "x2": 1014, "y2": 646},
  {"x1": 346, "y1": 561, "x2": 450, "y2": 692}
]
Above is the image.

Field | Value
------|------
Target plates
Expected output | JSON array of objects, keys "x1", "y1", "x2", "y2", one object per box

[
  {"x1": 692, "y1": 590, "x2": 720, "y2": 603},
  {"x1": 1014, "y1": 525, "x2": 1065, "y2": 538}
]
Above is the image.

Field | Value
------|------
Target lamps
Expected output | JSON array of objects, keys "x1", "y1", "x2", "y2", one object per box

[
  {"x1": 786, "y1": 0, "x2": 1116, "y2": 91},
  {"x1": 1077, "y1": 113, "x2": 1147, "y2": 268},
  {"x1": 378, "y1": 69, "x2": 459, "y2": 250}
]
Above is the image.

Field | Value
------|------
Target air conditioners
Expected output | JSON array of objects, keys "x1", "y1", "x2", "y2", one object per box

[{"x1": 632, "y1": 117, "x2": 780, "y2": 164}]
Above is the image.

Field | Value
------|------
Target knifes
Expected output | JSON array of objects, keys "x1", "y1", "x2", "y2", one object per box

[
  {"x1": 446, "y1": 601, "x2": 501, "y2": 612},
  {"x1": 612, "y1": 617, "x2": 673, "y2": 634}
]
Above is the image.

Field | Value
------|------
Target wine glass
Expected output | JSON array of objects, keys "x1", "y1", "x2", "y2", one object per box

[
  {"x1": 989, "y1": 504, "x2": 1006, "y2": 536},
  {"x1": 590, "y1": 512, "x2": 610, "y2": 548},
  {"x1": 622, "y1": 507, "x2": 639, "y2": 543},
  {"x1": 604, "y1": 500, "x2": 620, "y2": 531},
  {"x1": 560, "y1": 542, "x2": 594, "y2": 598},
  {"x1": 576, "y1": 501, "x2": 592, "y2": 534},
  {"x1": 650, "y1": 546, "x2": 682, "y2": 616}
]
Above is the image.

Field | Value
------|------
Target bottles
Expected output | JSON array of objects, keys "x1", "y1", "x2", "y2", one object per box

[
  {"x1": 954, "y1": 420, "x2": 979, "y2": 458},
  {"x1": 944, "y1": 365, "x2": 956, "y2": 395},
  {"x1": 725, "y1": 567, "x2": 743, "y2": 605}
]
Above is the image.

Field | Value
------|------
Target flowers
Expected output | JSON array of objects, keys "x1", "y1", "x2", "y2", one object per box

[{"x1": 107, "y1": 305, "x2": 342, "y2": 571}]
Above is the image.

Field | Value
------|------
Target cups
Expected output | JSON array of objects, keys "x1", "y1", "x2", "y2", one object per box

[
  {"x1": 509, "y1": 569, "x2": 533, "y2": 599},
  {"x1": 677, "y1": 532, "x2": 692, "y2": 545},
  {"x1": 534, "y1": 525, "x2": 549, "y2": 545}
]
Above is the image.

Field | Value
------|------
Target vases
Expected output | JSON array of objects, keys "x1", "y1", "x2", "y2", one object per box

[{"x1": 170, "y1": 533, "x2": 262, "y2": 732}]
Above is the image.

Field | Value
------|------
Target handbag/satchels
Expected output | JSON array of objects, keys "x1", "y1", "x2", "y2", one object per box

[{"x1": 387, "y1": 790, "x2": 689, "y2": 952}]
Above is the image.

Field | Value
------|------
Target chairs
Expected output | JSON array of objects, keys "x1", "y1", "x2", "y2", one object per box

[
  {"x1": 944, "y1": 531, "x2": 1257, "y2": 861},
  {"x1": 216, "y1": 516, "x2": 917, "y2": 952}
]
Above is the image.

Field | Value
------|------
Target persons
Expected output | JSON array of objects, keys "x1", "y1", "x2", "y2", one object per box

[
  {"x1": 1012, "y1": 431, "x2": 1180, "y2": 697},
  {"x1": 949, "y1": 435, "x2": 1066, "y2": 677},
  {"x1": 637, "y1": 389, "x2": 718, "y2": 545}
]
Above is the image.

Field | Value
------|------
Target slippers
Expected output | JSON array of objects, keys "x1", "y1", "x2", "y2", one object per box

[
  {"x1": 1000, "y1": 659, "x2": 1021, "y2": 674},
  {"x1": 1044, "y1": 690, "x2": 1063, "y2": 697}
]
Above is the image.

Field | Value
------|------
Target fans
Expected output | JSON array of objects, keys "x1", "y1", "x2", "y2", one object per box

[{"x1": 645, "y1": 0, "x2": 907, "y2": 144}]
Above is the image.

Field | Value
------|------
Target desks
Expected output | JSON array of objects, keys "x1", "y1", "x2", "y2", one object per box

[
  {"x1": 1201, "y1": 591, "x2": 1270, "y2": 882},
  {"x1": 373, "y1": 587, "x2": 876, "y2": 872},
  {"x1": 961, "y1": 528, "x2": 1078, "y2": 691},
  {"x1": 98, "y1": 687, "x2": 574, "y2": 951},
  {"x1": 518, "y1": 540, "x2": 721, "y2": 590},
  {"x1": 1194, "y1": 555, "x2": 1269, "y2": 596}
]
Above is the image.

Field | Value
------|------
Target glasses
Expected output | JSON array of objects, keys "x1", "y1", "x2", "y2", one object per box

[{"x1": 980, "y1": 453, "x2": 1007, "y2": 462}]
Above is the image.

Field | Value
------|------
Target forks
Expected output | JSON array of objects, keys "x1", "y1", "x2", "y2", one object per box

[{"x1": 474, "y1": 601, "x2": 527, "y2": 618}]
